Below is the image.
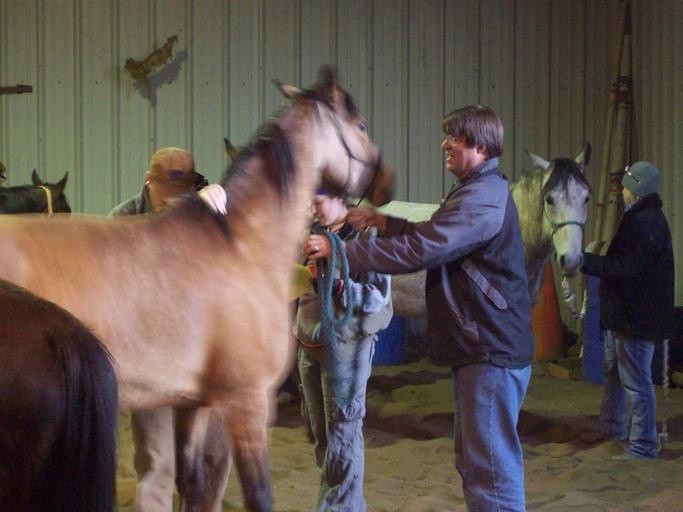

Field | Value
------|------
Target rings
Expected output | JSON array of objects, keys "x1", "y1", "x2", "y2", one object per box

[{"x1": 313, "y1": 245, "x2": 323, "y2": 251}]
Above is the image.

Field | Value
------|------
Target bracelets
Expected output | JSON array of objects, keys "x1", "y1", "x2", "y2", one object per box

[{"x1": 337, "y1": 279, "x2": 344, "y2": 296}]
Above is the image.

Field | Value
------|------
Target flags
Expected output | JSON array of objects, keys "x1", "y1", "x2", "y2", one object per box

[{"x1": 597, "y1": 1, "x2": 634, "y2": 257}]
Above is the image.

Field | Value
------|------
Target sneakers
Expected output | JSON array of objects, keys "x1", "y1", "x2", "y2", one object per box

[{"x1": 579, "y1": 428, "x2": 629, "y2": 445}]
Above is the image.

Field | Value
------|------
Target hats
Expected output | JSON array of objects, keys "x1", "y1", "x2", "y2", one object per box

[
  {"x1": 620, "y1": 160, "x2": 661, "y2": 199},
  {"x1": 147, "y1": 145, "x2": 197, "y2": 187}
]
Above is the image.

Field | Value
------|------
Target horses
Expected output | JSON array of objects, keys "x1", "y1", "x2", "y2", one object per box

[
  {"x1": 0, "y1": 168, "x2": 72, "y2": 213},
  {"x1": 0, "y1": 61, "x2": 398, "y2": 512},
  {"x1": 0, "y1": 279, "x2": 118, "y2": 512},
  {"x1": 344, "y1": 141, "x2": 593, "y2": 318}
]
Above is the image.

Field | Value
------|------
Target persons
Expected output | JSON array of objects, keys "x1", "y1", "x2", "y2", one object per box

[
  {"x1": 578, "y1": 157, "x2": 674, "y2": 459},
  {"x1": 304, "y1": 97, "x2": 533, "y2": 512},
  {"x1": 291, "y1": 183, "x2": 391, "y2": 512},
  {"x1": 103, "y1": 145, "x2": 211, "y2": 511}
]
